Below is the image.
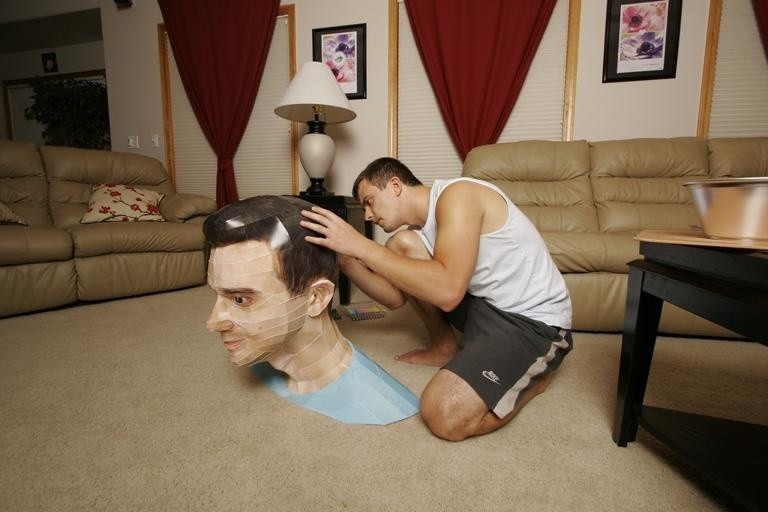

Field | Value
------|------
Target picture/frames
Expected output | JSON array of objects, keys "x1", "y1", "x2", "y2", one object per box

[
  {"x1": 312, "y1": 23, "x2": 366, "y2": 99},
  {"x1": 602, "y1": 0, "x2": 682, "y2": 82}
]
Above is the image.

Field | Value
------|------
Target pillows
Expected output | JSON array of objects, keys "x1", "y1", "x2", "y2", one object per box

[
  {"x1": 79, "y1": 183, "x2": 167, "y2": 224},
  {"x1": 0, "y1": 201, "x2": 29, "y2": 226}
]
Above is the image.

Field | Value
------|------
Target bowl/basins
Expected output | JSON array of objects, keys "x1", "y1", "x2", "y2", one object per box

[{"x1": 682, "y1": 177, "x2": 768, "y2": 242}]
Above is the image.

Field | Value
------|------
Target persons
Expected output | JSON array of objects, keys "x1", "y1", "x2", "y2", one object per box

[
  {"x1": 202, "y1": 192, "x2": 423, "y2": 427},
  {"x1": 298, "y1": 153, "x2": 576, "y2": 444}
]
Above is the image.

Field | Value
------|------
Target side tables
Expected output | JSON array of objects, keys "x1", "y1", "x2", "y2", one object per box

[{"x1": 283, "y1": 195, "x2": 374, "y2": 306}]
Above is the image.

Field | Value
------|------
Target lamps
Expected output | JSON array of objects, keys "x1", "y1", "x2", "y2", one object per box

[{"x1": 274, "y1": 61, "x2": 357, "y2": 203}]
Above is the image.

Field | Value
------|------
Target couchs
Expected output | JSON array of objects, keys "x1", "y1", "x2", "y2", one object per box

[
  {"x1": 0, "y1": 139, "x2": 214, "y2": 319},
  {"x1": 463, "y1": 138, "x2": 768, "y2": 340}
]
